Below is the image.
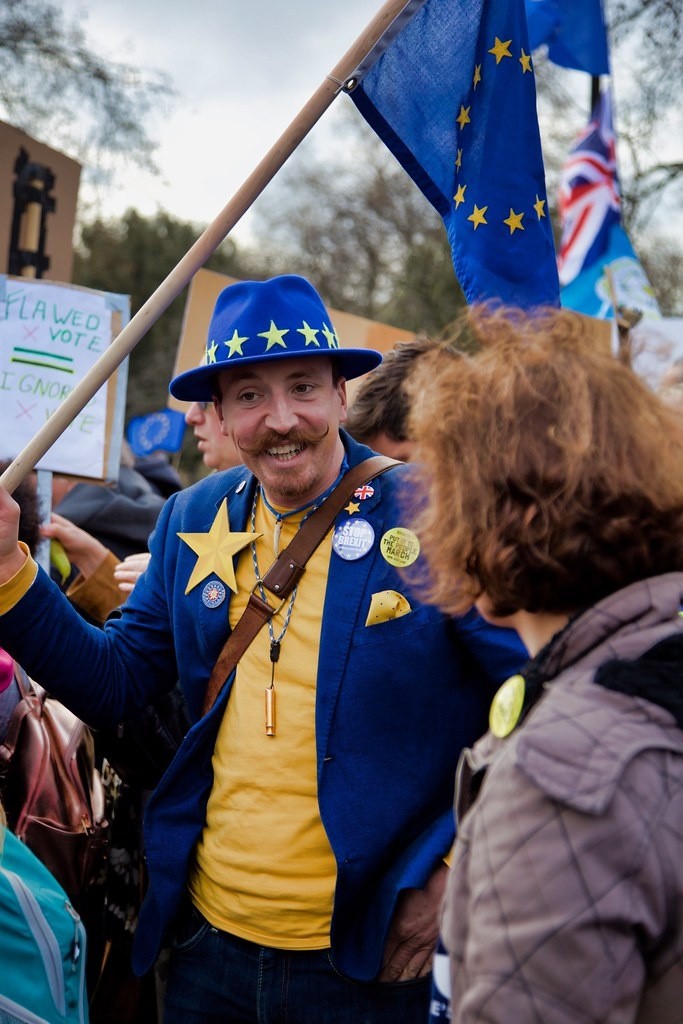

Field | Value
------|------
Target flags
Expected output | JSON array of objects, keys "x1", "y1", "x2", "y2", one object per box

[
  {"x1": 549, "y1": 87, "x2": 619, "y2": 282},
  {"x1": 547, "y1": 221, "x2": 660, "y2": 324},
  {"x1": 126, "y1": 408, "x2": 188, "y2": 458},
  {"x1": 352, "y1": 0, "x2": 560, "y2": 333}
]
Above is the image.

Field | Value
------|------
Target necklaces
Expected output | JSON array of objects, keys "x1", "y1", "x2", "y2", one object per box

[{"x1": 250, "y1": 454, "x2": 349, "y2": 734}]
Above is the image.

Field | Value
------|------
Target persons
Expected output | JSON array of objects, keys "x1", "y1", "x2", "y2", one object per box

[
  {"x1": 0, "y1": 275, "x2": 533, "y2": 1023},
  {"x1": 345, "y1": 338, "x2": 683, "y2": 1023},
  {"x1": 0, "y1": 348, "x2": 244, "y2": 1024}
]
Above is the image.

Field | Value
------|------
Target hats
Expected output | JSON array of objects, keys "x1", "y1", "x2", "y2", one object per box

[{"x1": 170, "y1": 276, "x2": 382, "y2": 404}]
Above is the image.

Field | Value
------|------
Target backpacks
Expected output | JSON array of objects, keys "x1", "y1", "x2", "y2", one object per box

[{"x1": 0, "y1": 660, "x2": 108, "y2": 915}]
